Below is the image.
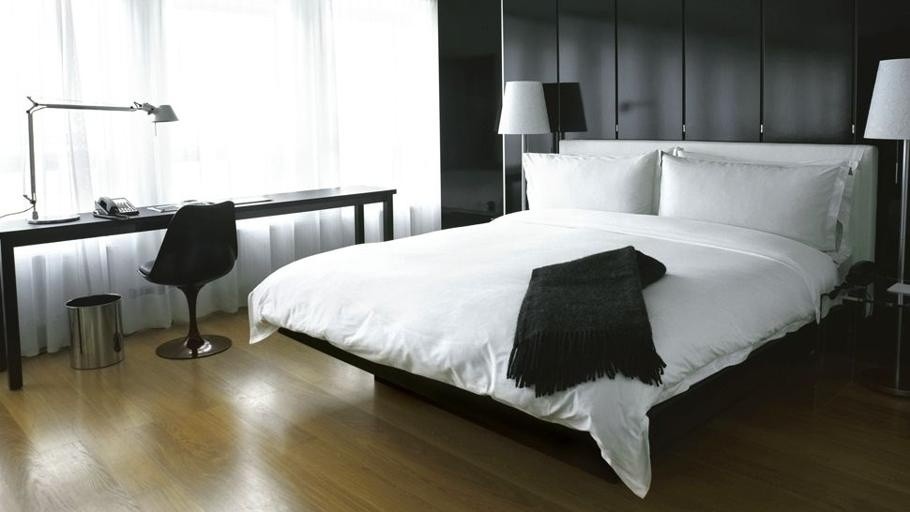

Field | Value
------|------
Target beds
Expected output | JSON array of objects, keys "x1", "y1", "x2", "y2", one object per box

[{"x1": 246, "y1": 138, "x2": 878, "y2": 500}]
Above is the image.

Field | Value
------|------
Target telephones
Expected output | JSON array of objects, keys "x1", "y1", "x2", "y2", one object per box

[{"x1": 95, "y1": 196, "x2": 139, "y2": 216}]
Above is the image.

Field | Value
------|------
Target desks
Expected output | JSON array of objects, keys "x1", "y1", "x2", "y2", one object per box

[{"x1": 0, "y1": 180, "x2": 396, "y2": 391}]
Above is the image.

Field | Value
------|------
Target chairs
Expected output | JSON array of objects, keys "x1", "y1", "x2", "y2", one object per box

[{"x1": 138, "y1": 201, "x2": 239, "y2": 360}]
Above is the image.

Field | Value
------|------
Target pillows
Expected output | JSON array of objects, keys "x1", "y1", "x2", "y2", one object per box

[
  {"x1": 522, "y1": 152, "x2": 659, "y2": 214},
  {"x1": 658, "y1": 152, "x2": 859, "y2": 251}
]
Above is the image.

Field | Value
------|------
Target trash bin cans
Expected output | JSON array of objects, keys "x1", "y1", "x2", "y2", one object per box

[{"x1": 64, "y1": 294, "x2": 125, "y2": 370}]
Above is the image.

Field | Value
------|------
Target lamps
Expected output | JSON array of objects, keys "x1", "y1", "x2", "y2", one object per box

[
  {"x1": 24, "y1": 96, "x2": 179, "y2": 224},
  {"x1": 496, "y1": 80, "x2": 552, "y2": 211},
  {"x1": 859, "y1": 57, "x2": 910, "y2": 396}
]
